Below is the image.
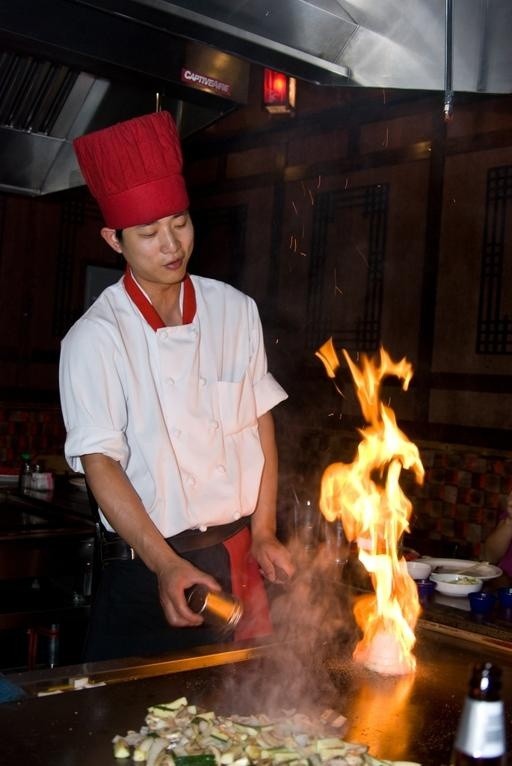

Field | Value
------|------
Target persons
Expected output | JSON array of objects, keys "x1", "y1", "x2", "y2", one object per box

[
  {"x1": 59, "y1": 209, "x2": 299, "y2": 653},
  {"x1": 481, "y1": 491, "x2": 512, "y2": 589}
]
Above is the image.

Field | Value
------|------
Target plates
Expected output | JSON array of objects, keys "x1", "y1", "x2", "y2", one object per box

[
  {"x1": 71, "y1": 478, "x2": 90, "y2": 492},
  {"x1": 0, "y1": 473, "x2": 19, "y2": 484}
]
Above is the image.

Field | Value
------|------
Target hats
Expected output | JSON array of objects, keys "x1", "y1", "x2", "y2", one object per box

[{"x1": 74, "y1": 101, "x2": 188, "y2": 228}]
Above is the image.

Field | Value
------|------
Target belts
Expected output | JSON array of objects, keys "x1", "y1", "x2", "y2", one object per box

[{"x1": 99, "y1": 515, "x2": 260, "y2": 561}]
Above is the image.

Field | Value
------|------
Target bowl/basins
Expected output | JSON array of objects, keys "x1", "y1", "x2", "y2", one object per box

[{"x1": 399, "y1": 547, "x2": 511, "y2": 616}]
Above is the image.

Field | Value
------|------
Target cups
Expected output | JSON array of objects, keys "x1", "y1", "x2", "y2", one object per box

[{"x1": 188, "y1": 586, "x2": 245, "y2": 643}]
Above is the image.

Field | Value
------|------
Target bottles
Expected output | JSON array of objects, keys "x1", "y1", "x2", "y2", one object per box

[
  {"x1": 20, "y1": 454, "x2": 40, "y2": 491},
  {"x1": 451, "y1": 663, "x2": 508, "y2": 766}
]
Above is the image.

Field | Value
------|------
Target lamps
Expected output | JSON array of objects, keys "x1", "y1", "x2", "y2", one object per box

[{"x1": 263, "y1": 68, "x2": 296, "y2": 115}]
